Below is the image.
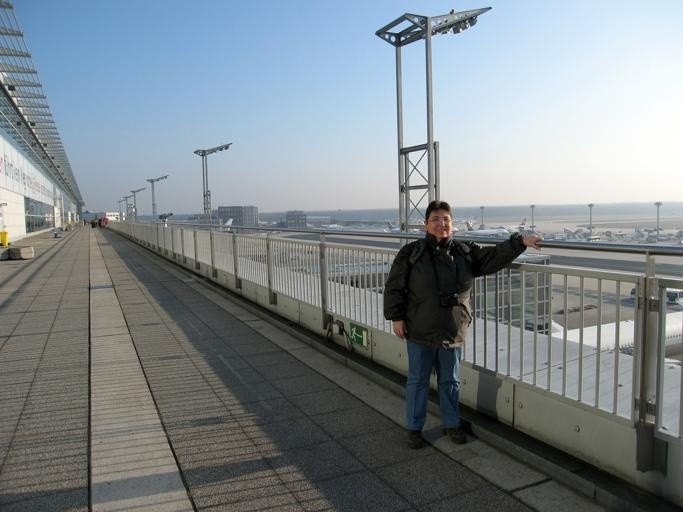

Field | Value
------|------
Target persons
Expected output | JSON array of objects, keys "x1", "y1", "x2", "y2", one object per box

[{"x1": 384, "y1": 202, "x2": 544, "y2": 448}]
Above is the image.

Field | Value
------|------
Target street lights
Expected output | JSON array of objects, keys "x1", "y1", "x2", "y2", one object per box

[
  {"x1": 374, "y1": 6, "x2": 493, "y2": 232},
  {"x1": 477, "y1": 204, "x2": 485, "y2": 230},
  {"x1": 585, "y1": 202, "x2": 595, "y2": 238},
  {"x1": 193, "y1": 141, "x2": 232, "y2": 220},
  {"x1": 146, "y1": 173, "x2": 170, "y2": 221},
  {"x1": 652, "y1": 201, "x2": 663, "y2": 244},
  {"x1": 122, "y1": 194, "x2": 133, "y2": 213},
  {"x1": 130, "y1": 187, "x2": 146, "y2": 220},
  {"x1": 528, "y1": 203, "x2": 536, "y2": 231},
  {"x1": 117, "y1": 199, "x2": 125, "y2": 222}
]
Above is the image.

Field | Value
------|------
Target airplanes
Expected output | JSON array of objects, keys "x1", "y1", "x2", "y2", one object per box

[{"x1": 384, "y1": 220, "x2": 526, "y2": 238}]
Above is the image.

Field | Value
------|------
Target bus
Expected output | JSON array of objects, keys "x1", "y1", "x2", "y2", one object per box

[
  {"x1": 630, "y1": 285, "x2": 683, "y2": 304},
  {"x1": 587, "y1": 236, "x2": 600, "y2": 243}
]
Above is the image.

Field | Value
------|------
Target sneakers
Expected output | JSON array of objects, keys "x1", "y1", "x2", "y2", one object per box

[
  {"x1": 408, "y1": 427, "x2": 421, "y2": 441},
  {"x1": 441, "y1": 426, "x2": 467, "y2": 444}
]
